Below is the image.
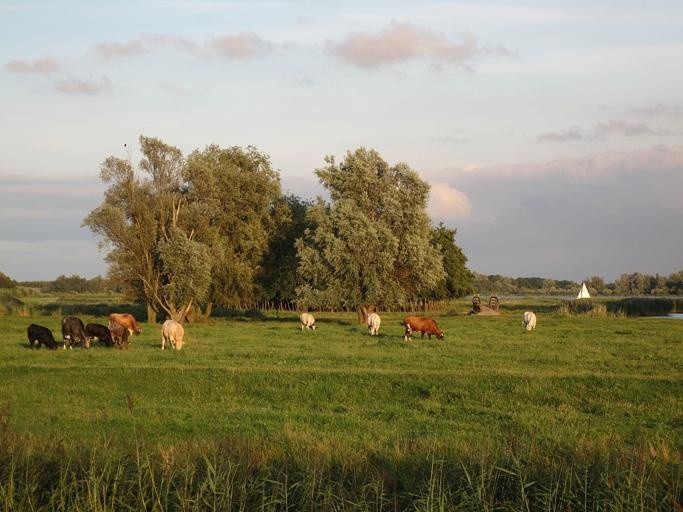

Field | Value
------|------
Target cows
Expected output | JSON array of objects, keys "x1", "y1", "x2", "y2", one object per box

[
  {"x1": 61, "y1": 312, "x2": 141, "y2": 350},
  {"x1": 522, "y1": 311, "x2": 537, "y2": 331},
  {"x1": 367, "y1": 313, "x2": 381, "y2": 336},
  {"x1": 299, "y1": 313, "x2": 318, "y2": 332},
  {"x1": 161, "y1": 319, "x2": 186, "y2": 351},
  {"x1": 27, "y1": 324, "x2": 59, "y2": 351},
  {"x1": 403, "y1": 316, "x2": 444, "y2": 341}
]
